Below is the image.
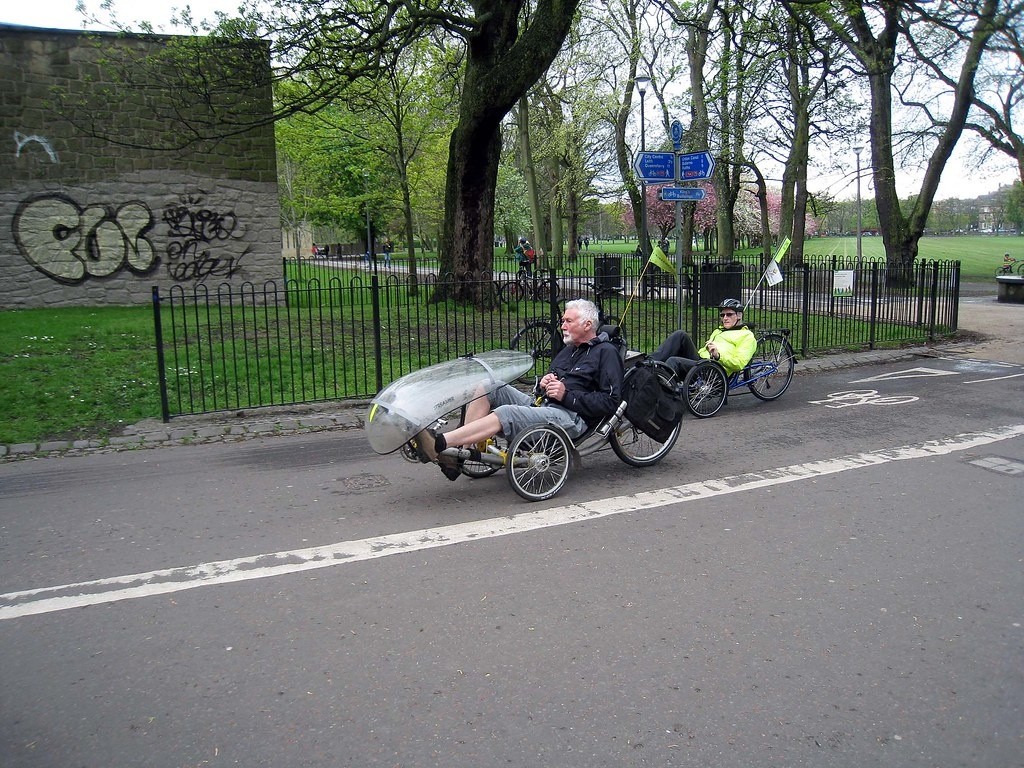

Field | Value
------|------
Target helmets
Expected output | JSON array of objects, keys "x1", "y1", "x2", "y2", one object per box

[{"x1": 718, "y1": 299, "x2": 744, "y2": 311}]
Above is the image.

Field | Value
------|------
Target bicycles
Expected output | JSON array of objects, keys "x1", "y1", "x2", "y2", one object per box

[
  {"x1": 509, "y1": 283, "x2": 627, "y2": 385},
  {"x1": 683, "y1": 323, "x2": 798, "y2": 418},
  {"x1": 365, "y1": 324, "x2": 683, "y2": 501},
  {"x1": 995, "y1": 260, "x2": 1024, "y2": 277},
  {"x1": 499, "y1": 267, "x2": 560, "y2": 304}
]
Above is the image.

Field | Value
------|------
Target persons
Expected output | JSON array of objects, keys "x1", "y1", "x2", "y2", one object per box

[
  {"x1": 311, "y1": 242, "x2": 324, "y2": 254},
  {"x1": 364, "y1": 251, "x2": 372, "y2": 268},
  {"x1": 412, "y1": 299, "x2": 624, "y2": 481},
  {"x1": 577, "y1": 236, "x2": 583, "y2": 251},
  {"x1": 643, "y1": 298, "x2": 758, "y2": 389},
  {"x1": 515, "y1": 238, "x2": 532, "y2": 280},
  {"x1": 324, "y1": 244, "x2": 329, "y2": 256},
  {"x1": 382, "y1": 238, "x2": 392, "y2": 268},
  {"x1": 635, "y1": 245, "x2": 643, "y2": 257},
  {"x1": 583, "y1": 236, "x2": 590, "y2": 251},
  {"x1": 1002, "y1": 253, "x2": 1016, "y2": 274},
  {"x1": 336, "y1": 243, "x2": 344, "y2": 262}
]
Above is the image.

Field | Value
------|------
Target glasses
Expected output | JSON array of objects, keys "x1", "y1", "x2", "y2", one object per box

[{"x1": 720, "y1": 313, "x2": 736, "y2": 317}]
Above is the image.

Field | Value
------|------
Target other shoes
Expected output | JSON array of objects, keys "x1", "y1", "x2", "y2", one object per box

[
  {"x1": 437, "y1": 455, "x2": 462, "y2": 481},
  {"x1": 415, "y1": 428, "x2": 439, "y2": 464}
]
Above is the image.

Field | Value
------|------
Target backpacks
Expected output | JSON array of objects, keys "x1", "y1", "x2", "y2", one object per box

[{"x1": 520, "y1": 242, "x2": 535, "y2": 263}]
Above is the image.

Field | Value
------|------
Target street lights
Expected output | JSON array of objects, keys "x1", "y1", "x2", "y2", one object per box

[
  {"x1": 362, "y1": 167, "x2": 372, "y2": 257},
  {"x1": 854, "y1": 144, "x2": 865, "y2": 267}
]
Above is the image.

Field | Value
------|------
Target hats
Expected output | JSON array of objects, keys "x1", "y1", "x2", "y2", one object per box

[{"x1": 520, "y1": 238, "x2": 526, "y2": 244}]
